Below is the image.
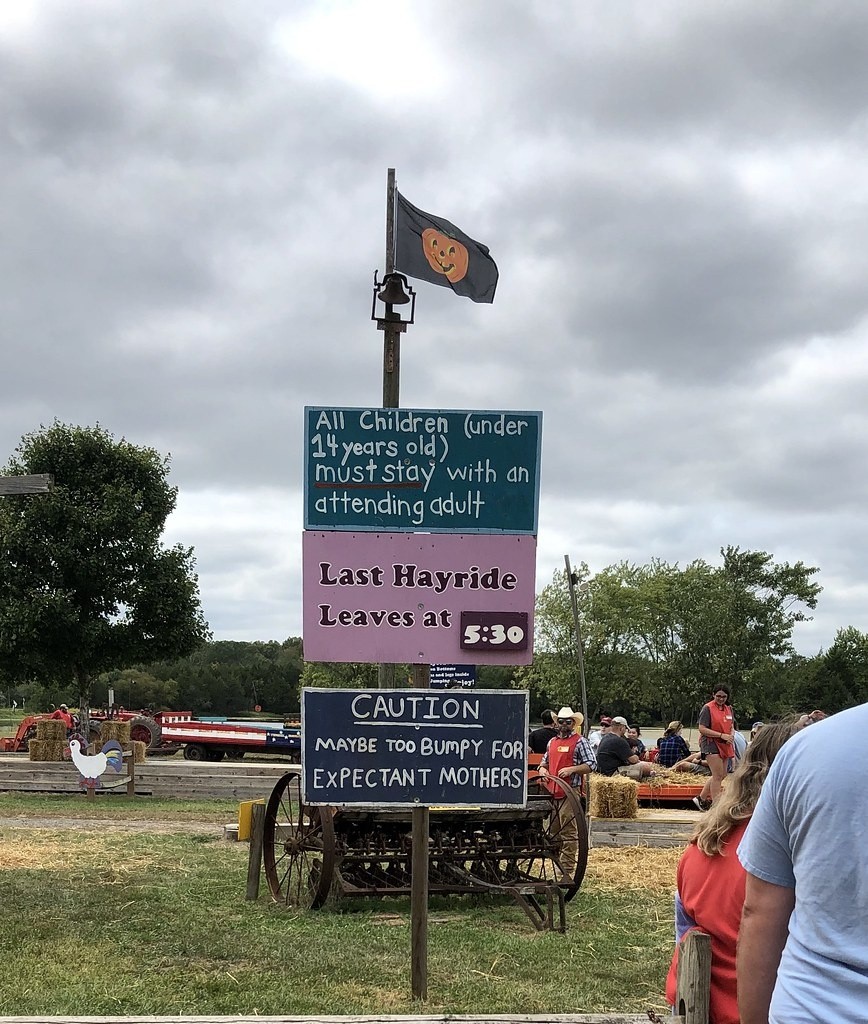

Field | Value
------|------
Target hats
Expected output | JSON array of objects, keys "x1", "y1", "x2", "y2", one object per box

[
  {"x1": 60, "y1": 704, "x2": 69, "y2": 709},
  {"x1": 600, "y1": 715, "x2": 612, "y2": 725},
  {"x1": 612, "y1": 716, "x2": 631, "y2": 732},
  {"x1": 550, "y1": 707, "x2": 584, "y2": 726},
  {"x1": 750, "y1": 722, "x2": 765, "y2": 732}
]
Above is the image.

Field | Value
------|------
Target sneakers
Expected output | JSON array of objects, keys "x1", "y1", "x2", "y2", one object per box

[{"x1": 692, "y1": 795, "x2": 706, "y2": 812}]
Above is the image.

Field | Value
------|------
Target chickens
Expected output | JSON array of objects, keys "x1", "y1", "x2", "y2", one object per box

[{"x1": 64, "y1": 733, "x2": 122, "y2": 789}]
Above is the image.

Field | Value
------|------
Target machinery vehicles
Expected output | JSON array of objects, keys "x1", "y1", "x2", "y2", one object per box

[{"x1": 0, "y1": 687, "x2": 186, "y2": 756}]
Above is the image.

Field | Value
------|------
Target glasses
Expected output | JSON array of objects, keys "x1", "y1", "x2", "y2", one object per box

[
  {"x1": 601, "y1": 724, "x2": 610, "y2": 727},
  {"x1": 716, "y1": 695, "x2": 727, "y2": 698},
  {"x1": 557, "y1": 718, "x2": 571, "y2": 725}
]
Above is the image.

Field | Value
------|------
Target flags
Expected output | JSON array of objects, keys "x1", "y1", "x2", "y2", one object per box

[{"x1": 392, "y1": 191, "x2": 498, "y2": 303}]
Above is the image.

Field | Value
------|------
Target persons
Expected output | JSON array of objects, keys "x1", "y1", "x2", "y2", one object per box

[
  {"x1": 735, "y1": 701, "x2": 868, "y2": 1024},
  {"x1": 526, "y1": 683, "x2": 764, "y2": 875},
  {"x1": 51, "y1": 703, "x2": 75, "y2": 739},
  {"x1": 664, "y1": 710, "x2": 828, "y2": 1024}
]
